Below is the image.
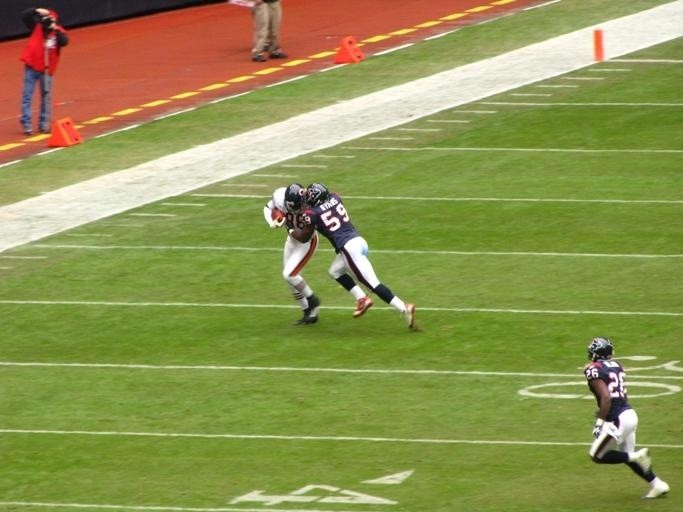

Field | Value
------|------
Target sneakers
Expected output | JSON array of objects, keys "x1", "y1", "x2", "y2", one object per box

[
  {"x1": 404, "y1": 303, "x2": 415, "y2": 328},
  {"x1": 296, "y1": 316, "x2": 319, "y2": 323},
  {"x1": 252, "y1": 54, "x2": 266, "y2": 61},
  {"x1": 40, "y1": 129, "x2": 50, "y2": 133},
  {"x1": 269, "y1": 52, "x2": 287, "y2": 58},
  {"x1": 352, "y1": 295, "x2": 373, "y2": 318},
  {"x1": 637, "y1": 448, "x2": 651, "y2": 474},
  {"x1": 643, "y1": 481, "x2": 671, "y2": 498},
  {"x1": 23, "y1": 128, "x2": 32, "y2": 134}
]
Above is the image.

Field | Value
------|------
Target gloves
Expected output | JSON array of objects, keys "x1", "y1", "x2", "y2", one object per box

[{"x1": 593, "y1": 425, "x2": 602, "y2": 438}]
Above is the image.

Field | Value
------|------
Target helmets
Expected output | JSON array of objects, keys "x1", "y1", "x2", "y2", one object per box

[
  {"x1": 283, "y1": 182, "x2": 305, "y2": 211},
  {"x1": 588, "y1": 337, "x2": 614, "y2": 361},
  {"x1": 306, "y1": 183, "x2": 329, "y2": 207}
]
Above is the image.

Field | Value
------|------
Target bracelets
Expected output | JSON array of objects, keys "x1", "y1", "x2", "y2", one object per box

[
  {"x1": 596, "y1": 418, "x2": 604, "y2": 427},
  {"x1": 289, "y1": 229, "x2": 294, "y2": 235}
]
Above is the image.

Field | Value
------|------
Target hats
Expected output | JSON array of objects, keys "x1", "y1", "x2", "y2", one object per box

[{"x1": 49, "y1": 10, "x2": 59, "y2": 19}]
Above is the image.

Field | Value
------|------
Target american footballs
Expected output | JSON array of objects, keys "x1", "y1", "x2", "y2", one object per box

[{"x1": 272, "y1": 208, "x2": 283, "y2": 222}]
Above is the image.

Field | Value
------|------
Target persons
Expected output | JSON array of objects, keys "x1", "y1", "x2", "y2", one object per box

[
  {"x1": 584, "y1": 338, "x2": 669, "y2": 499},
  {"x1": 285, "y1": 181, "x2": 415, "y2": 330},
  {"x1": 20, "y1": 7, "x2": 69, "y2": 135},
  {"x1": 264, "y1": 187, "x2": 321, "y2": 325},
  {"x1": 250, "y1": 0, "x2": 287, "y2": 62}
]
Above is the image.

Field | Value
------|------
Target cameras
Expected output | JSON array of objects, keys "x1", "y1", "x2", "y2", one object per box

[{"x1": 40, "y1": 15, "x2": 51, "y2": 33}]
